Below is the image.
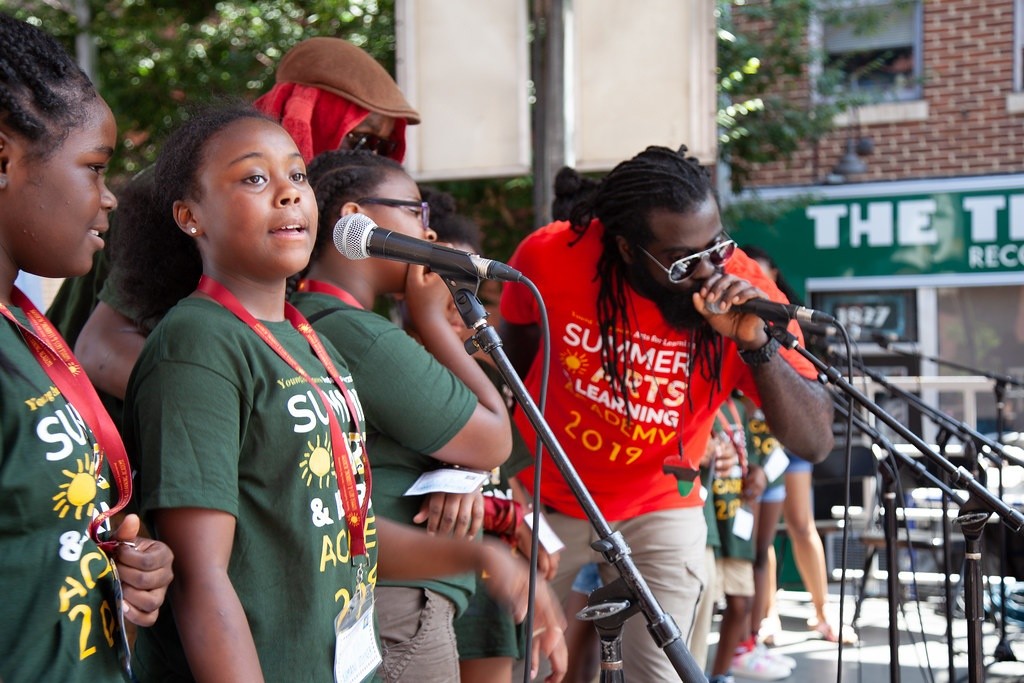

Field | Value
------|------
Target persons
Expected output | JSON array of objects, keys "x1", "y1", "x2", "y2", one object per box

[
  {"x1": 118, "y1": 105, "x2": 570, "y2": 683},
  {"x1": 290, "y1": 146, "x2": 515, "y2": 683},
  {"x1": 47, "y1": 36, "x2": 420, "y2": 433},
  {"x1": 380, "y1": 185, "x2": 558, "y2": 682},
  {"x1": 700, "y1": 238, "x2": 857, "y2": 683},
  {"x1": 498, "y1": 143, "x2": 839, "y2": 683},
  {"x1": 1, "y1": 11, "x2": 173, "y2": 683}
]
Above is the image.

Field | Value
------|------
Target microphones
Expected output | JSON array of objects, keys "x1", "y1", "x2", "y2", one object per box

[
  {"x1": 705, "y1": 291, "x2": 898, "y2": 361},
  {"x1": 331, "y1": 213, "x2": 522, "y2": 284}
]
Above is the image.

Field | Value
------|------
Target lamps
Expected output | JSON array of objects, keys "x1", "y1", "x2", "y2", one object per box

[{"x1": 831, "y1": 109, "x2": 874, "y2": 175}]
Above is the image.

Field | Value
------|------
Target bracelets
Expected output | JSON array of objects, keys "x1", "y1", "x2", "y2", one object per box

[{"x1": 737, "y1": 327, "x2": 780, "y2": 365}]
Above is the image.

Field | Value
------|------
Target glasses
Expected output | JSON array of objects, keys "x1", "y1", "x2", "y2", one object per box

[
  {"x1": 345, "y1": 131, "x2": 397, "y2": 156},
  {"x1": 356, "y1": 197, "x2": 430, "y2": 230},
  {"x1": 636, "y1": 230, "x2": 738, "y2": 283}
]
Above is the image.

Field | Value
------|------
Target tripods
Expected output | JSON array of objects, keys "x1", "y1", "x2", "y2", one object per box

[{"x1": 877, "y1": 341, "x2": 1024, "y2": 682}]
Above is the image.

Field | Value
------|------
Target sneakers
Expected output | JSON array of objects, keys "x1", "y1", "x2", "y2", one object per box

[
  {"x1": 730, "y1": 652, "x2": 791, "y2": 679},
  {"x1": 752, "y1": 647, "x2": 796, "y2": 668}
]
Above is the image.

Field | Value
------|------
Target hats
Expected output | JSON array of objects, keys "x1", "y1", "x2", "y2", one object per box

[{"x1": 276, "y1": 37, "x2": 422, "y2": 126}]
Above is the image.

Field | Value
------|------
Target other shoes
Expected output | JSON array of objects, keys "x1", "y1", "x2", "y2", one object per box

[{"x1": 808, "y1": 617, "x2": 860, "y2": 648}]
